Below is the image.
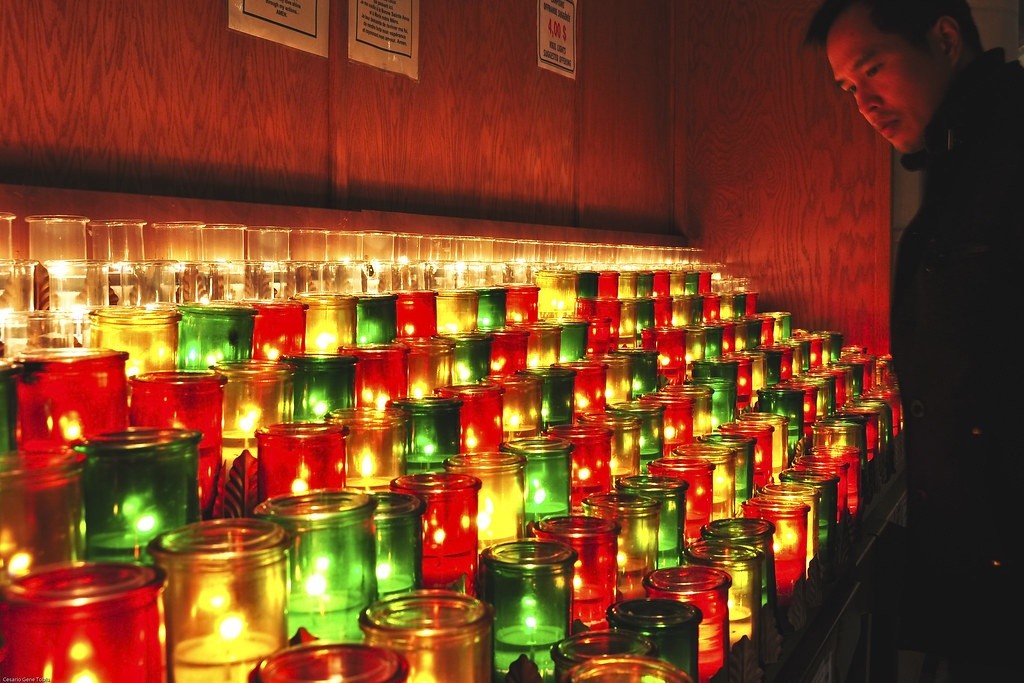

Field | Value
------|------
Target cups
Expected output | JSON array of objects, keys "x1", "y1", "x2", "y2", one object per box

[{"x1": 0, "y1": 212, "x2": 902, "y2": 683}]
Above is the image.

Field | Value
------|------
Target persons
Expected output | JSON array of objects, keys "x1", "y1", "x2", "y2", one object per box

[{"x1": 803, "y1": 0, "x2": 1024, "y2": 683}]
